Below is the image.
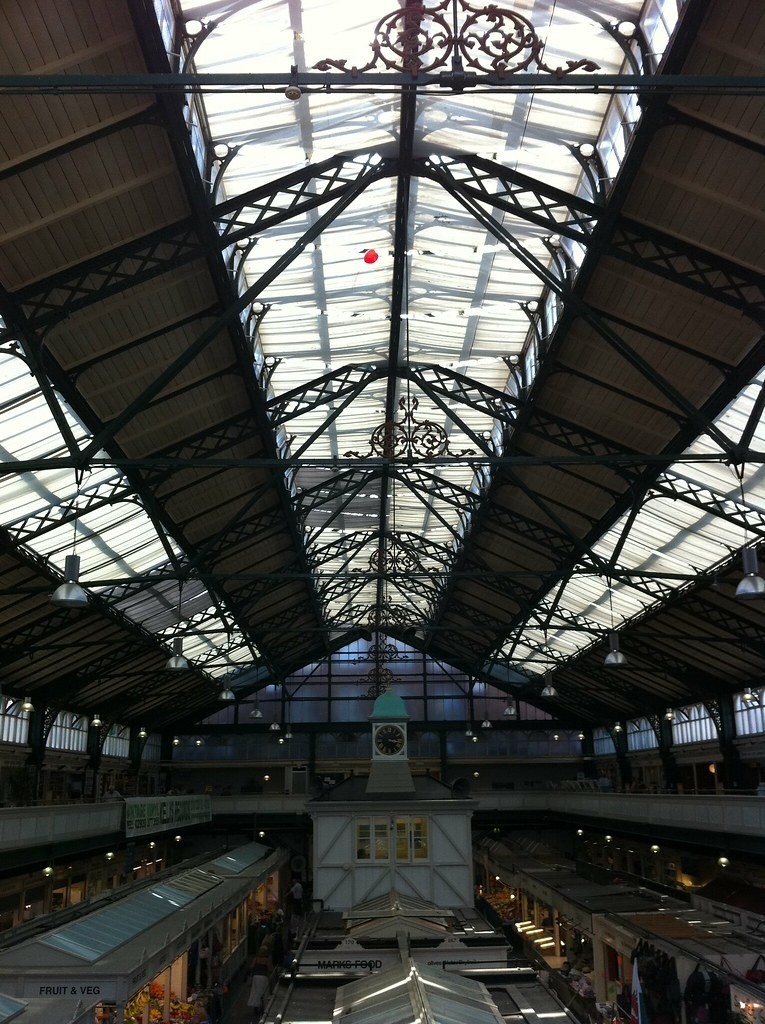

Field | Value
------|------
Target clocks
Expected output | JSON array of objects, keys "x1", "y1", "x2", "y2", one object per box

[{"x1": 373, "y1": 724, "x2": 405, "y2": 756}]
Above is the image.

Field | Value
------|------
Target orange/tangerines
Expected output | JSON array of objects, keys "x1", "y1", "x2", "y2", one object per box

[{"x1": 149, "y1": 981, "x2": 165, "y2": 999}]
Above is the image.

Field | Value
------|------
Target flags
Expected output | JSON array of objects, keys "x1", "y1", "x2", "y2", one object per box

[{"x1": 630, "y1": 960, "x2": 648, "y2": 1024}]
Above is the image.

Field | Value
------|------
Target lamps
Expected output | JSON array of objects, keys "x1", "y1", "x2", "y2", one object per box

[
  {"x1": 248, "y1": 663, "x2": 294, "y2": 744},
  {"x1": 733, "y1": 459, "x2": 765, "y2": 600},
  {"x1": 218, "y1": 632, "x2": 236, "y2": 701},
  {"x1": 175, "y1": 836, "x2": 181, "y2": 842},
  {"x1": 105, "y1": 851, "x2": 115, "y2": 860},
  {"x1": 263, "y1": 775, "x2": 269, "y2": 781},
  {"x1": 258, "y1": 831, "x2": 265, "y2": 837},
  {"x1": 539, "y1": 629, "x2": 556, "y2": 696},
  {"x1": 577, "y1": 829, "x2": 730, "y2": 872},
  {"x1": 553, "y1": 685, "x2": 758, "y2": 741},
  {"x1": 165, "y1": 580, "x2": 189, "y2": 671},
  {"x1": 49, "y1": 483, "x2": 86, "y2": 606},
  {"x1": 43, "y1": 866, "x2": 54, "y2": 877},
  {"x1": 463, "y1": 657, "x2": 518, "y2": 744},
  {"x1": 603, "y1": 573, "x2": 628, "y2": 667},
  {"x1": 473, "y1": 772, "x2": 479, "y2": 777},
  {"x1": 20, "y1": 696, "x2": 202, "y2": 750}
]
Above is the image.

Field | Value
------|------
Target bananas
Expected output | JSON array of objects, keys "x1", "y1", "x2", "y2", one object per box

[{"x1": 123, "y1": 990, "x2": 190, "y2": 1015}]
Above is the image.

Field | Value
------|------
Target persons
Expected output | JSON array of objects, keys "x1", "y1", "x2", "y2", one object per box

[
  {"x1": 561, "y1": 961, "x2": 592, "y2": 986},
  {"x1": 285, "y1": 879, "x2": 303, "y2": 916},
  {"x1": 600, "y1": 772, "x2": 615, "y2": 793},
  {"x1": 248, "y1": 910, "x2": 292, "y2": 968},
  {"x1": 167, "y1": 786, "x2": 175, "y2": 794},
  {"x1": 103, "y1": 784, "x2": 121, "y2": 802},
  {"x1": 631, "y1": 776, "x2": 646, "y2": 794},
  {"x1": 250, "y1": 946, "x2": 271, "y2": 1016},
  {"x1": 206, "y1": 981, "x2": 224, "y2": 1024}
]
